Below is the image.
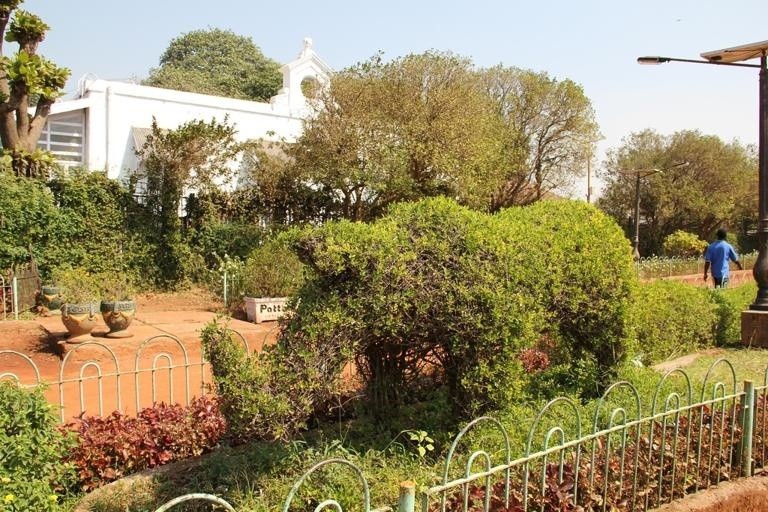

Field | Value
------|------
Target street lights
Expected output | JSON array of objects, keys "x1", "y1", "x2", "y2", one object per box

[
  {"x1": 634, "y1": 162, "x2": 690, "y2": 263},
  {"x1": 637, "y1": 57, "x2": 768, "y2": 311}
]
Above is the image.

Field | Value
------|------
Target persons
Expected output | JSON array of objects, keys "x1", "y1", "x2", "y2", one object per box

[{"x1": 703, "y1": 229, "x2": 744, "y2": 289}]
